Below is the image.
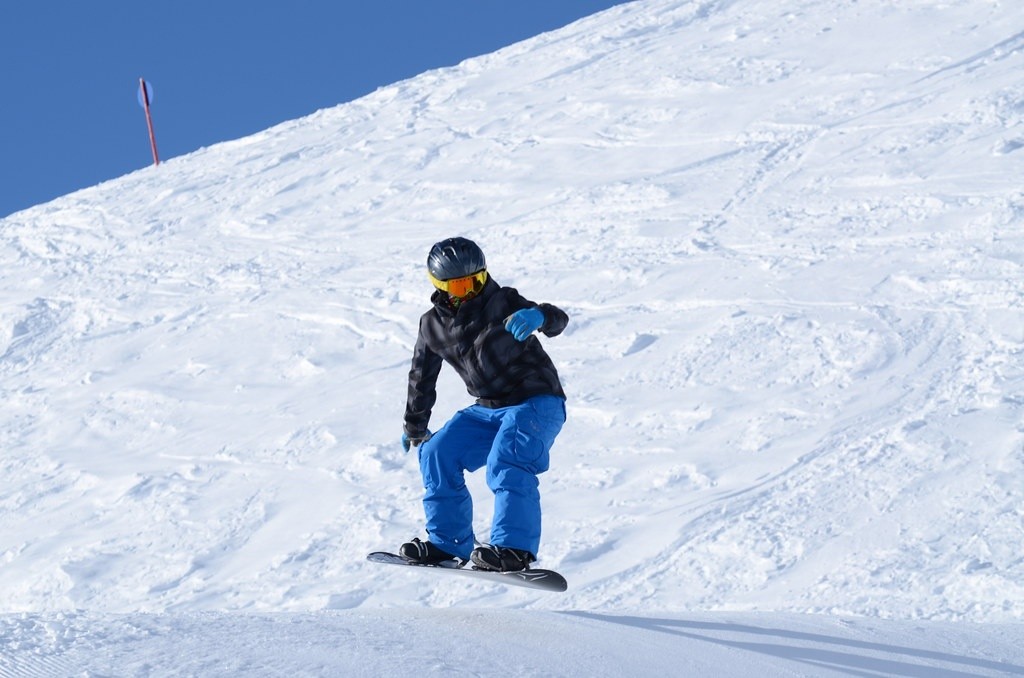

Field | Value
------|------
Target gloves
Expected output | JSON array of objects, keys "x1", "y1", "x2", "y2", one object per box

[
  {"x1": 401, "y1": 429, "x2": 432, "y2": 453},
  {"x1": 503, "y1": 307, "x2": 545, "y2": 342}
]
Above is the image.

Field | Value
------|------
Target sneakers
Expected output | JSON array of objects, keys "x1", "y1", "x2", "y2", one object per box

[
  {"x1": 400, "y1": 538, "x2": 471, "y2": 568},
  {"x1": 470, "y1": 543, "x2": 533, "y2": 574}
]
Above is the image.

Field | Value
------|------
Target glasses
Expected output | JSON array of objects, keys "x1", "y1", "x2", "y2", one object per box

[{"x1": 448, "y1": 270, "x2": 488, "y2": 298}]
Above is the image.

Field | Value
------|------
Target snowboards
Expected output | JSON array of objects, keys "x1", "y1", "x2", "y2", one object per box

[{"x1": 365, "y1": 550, "x2": 570, "y2": 594}]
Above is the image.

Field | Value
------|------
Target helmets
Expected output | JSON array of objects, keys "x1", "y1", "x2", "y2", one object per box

[{"x1": 427, "y1": 237, "x2": 487, "y2": 281}]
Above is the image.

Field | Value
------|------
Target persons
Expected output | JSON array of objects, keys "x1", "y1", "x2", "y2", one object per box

[{"x1": 401, "y1": 238, "x2": 570, "y2": 573}]
